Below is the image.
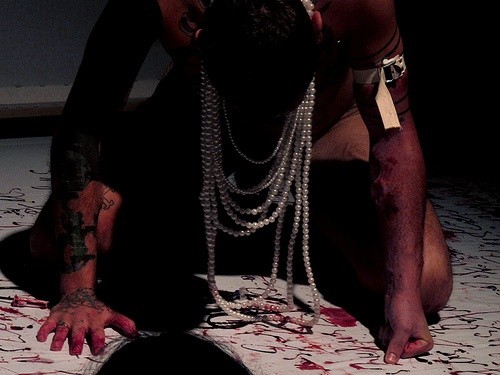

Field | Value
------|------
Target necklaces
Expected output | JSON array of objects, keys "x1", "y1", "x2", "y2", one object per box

[{"x1": 197, "y1": 0, "x2": 320, "y2": 328}]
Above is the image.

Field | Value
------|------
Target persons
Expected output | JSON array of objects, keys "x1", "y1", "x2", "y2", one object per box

[{"x1": 29, "y1": 0, "x2": 456, "y2": 365}]
